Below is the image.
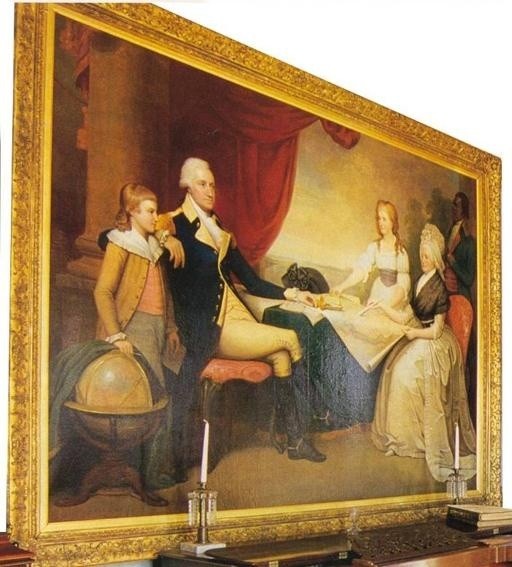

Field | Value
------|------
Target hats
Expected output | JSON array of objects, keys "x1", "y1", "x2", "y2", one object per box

[{"x1": 420, "y1": 223, "x2": 445, "y2": 256}]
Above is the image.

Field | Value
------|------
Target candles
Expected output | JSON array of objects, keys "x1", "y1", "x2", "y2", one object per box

[
  {"x1": 453, "y1": 420, "x2": 459, "y2": 468},
  {"x1": 199, "y1": 417, "x2": 211, "y2": 485}
]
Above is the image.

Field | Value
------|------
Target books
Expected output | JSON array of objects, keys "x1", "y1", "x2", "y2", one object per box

[{"x1": 447, "y1": 503, "x2": 512, "y2": 528}]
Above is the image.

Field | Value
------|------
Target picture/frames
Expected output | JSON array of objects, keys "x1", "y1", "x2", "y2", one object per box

[{"x1": 7, "y1": 2, "x2": 501, "y2": 567}]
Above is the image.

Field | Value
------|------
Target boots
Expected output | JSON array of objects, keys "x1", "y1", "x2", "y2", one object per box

[
  {"x1": 292, "y1": 356, "x2": 352, "y2": 432},
  {"x1": 142, "y1": 488, "x2": 169, "y2": 507},
  {"x1": 274, "y1": 375, "x2": 326, "y2": 463}
]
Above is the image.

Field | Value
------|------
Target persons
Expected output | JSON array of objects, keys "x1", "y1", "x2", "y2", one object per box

[
  {"x1": 93, "y1": 183, "x2": 190, "y2": 492},
  {"x1": 98, "y1": 157, "x2": 352, "y2": 463}
]
